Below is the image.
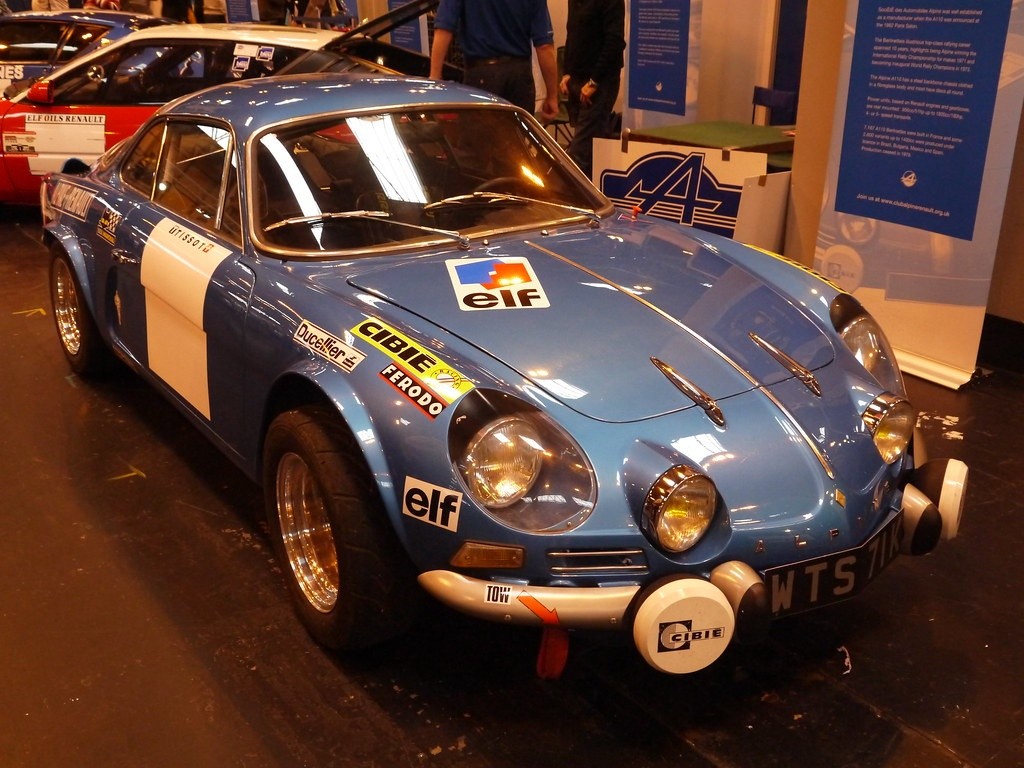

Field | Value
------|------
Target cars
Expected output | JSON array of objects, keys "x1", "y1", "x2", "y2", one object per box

[
  {"x1": 0, "y1": 0, "x2": 472, "y2": 211},
  {"x1": 0, "y1": 11, "x2": 270, "y2": 102},
  {"x1": 40, "y1": 73, "x2": 970, "y2": 682}
]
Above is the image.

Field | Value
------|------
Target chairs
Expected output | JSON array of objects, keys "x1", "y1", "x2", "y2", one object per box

[
  {"x1": 752, "y1": 86, "x2": 797, "y2": 126},
  {"x1": 535, "y1": 46, "x2": 573, "y2": 145}
]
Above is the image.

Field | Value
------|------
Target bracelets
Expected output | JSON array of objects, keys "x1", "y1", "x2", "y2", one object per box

[{"x1": 589, "y1": 79, "x2": 598, "y2": 87}]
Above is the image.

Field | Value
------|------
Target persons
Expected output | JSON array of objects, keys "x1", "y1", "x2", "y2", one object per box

[
  {"x1": 559, "y1": 0, "x2": 627, "y2": 180},
  {"x1": 427, "y1": 0, "x2": 559, "y2": 181},
  {"x1": 150, "y1": 0, "x2": 229, "y2": 24}
]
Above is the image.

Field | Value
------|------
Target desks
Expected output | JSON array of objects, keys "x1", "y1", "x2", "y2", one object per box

[{"x1": 628, "y1": 119, "x2": 794, "y2": 171}]
